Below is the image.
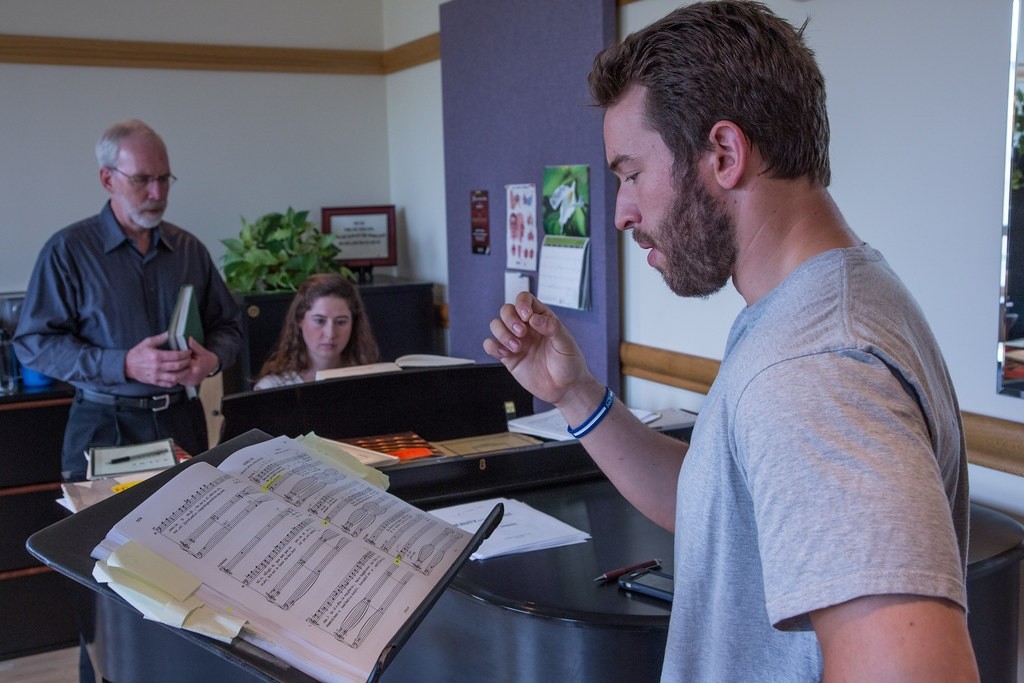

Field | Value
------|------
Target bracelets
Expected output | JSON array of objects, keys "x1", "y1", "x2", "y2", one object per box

[
  {"x1": 567, "y1": 386, "x2": 615, "y2": 439},
  {"x1": 207, "y1": 352, "x2": 220, "y2": 377}
]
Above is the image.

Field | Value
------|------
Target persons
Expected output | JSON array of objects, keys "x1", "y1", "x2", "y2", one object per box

[
  {"x1": 10, "y1": 118, "x2": 245, "y2": 483},
  {"x1": 253, "y1": 272, "x2": 380, "y2": 392},
  {"x1": 482, "y1": 0, "x2": 982, "y2": 683}
]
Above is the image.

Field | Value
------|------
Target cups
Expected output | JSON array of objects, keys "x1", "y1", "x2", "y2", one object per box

[
  {"x1": 21, "y1": 365, "x2": 54, "y2": 387},
  {"x1": 0, "y1": 342, "x2": 18, "y2": 396}
]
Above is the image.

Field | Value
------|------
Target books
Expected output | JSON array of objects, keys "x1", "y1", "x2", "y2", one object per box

[
  {"x1": 508, "y1": 406, "x2": 662, "y2": 442},
  {"x1": 56, "y1": 437, "x2": 193, "y2": 515},
  {"x1": 90, "y1": 433, "x2": 474, "y2": 683},
  {"x1": 314, "y1": 354, "x2": 476, "y2": 380},
  {"x1": 168, "y1": 284, "x2": 204, "y2": 400}
]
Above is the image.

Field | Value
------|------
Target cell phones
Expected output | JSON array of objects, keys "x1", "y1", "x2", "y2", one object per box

[{"x1": 618, "y1": 568, "x2": 675, "y2": 602}]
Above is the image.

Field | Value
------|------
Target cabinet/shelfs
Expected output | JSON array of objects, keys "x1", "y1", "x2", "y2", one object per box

[{"x1": 0, "y1": 381, "x2": 99, "y2": 659}]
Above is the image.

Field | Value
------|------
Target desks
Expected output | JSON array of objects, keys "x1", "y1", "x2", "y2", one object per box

[{"x1": 61, "y1": 406, "x2": 1024, "y2": 683}]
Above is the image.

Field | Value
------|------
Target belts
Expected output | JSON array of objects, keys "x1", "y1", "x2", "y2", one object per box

[{"x1": 75, "y1": 387, "x2": 183, "y2": 412}]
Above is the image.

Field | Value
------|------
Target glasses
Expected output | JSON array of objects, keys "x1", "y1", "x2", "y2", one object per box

[{"x1": 110, "y1": 167, "x2": 177, "y2": 190}]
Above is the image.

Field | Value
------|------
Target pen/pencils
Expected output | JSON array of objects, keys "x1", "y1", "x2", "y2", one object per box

[
  {"x1": 593, "y1": 558, "x2": 663, "y2": 582},
  {"x1": 105, "y1": 449, "x2": 168, "y2": 464}
]
